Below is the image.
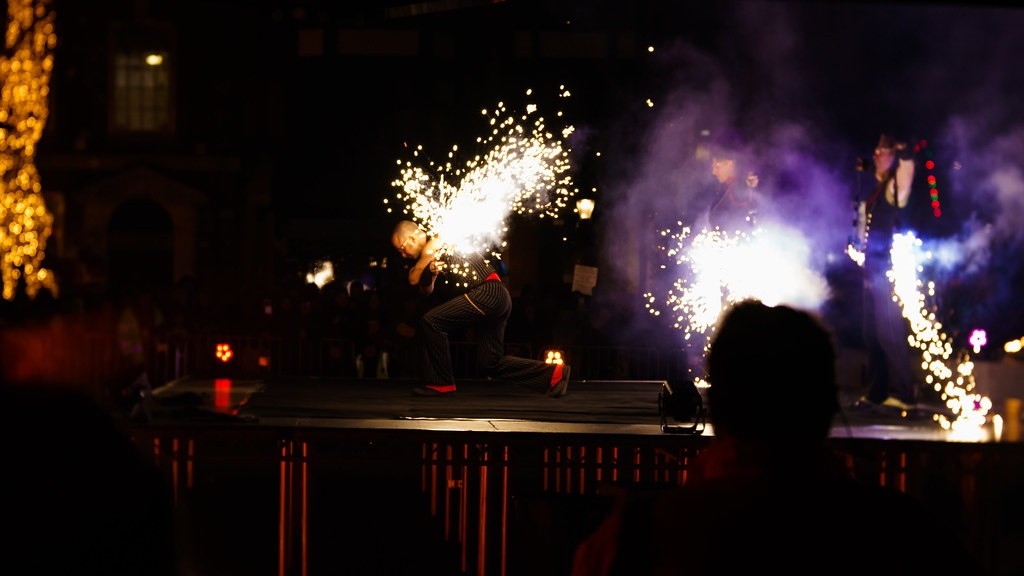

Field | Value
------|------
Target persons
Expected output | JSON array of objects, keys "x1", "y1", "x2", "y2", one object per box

[
  {"x1": 0, "y1": 381, "x2": 178, "y2": 576},
  {"x1": 391, "y1": 221, "x2": 571, "y2": 397},
  {"x1": 576, "y1": 301, "x2": 969, "y2": 576},
  {"x1": 846, "y1": 91, "x2": 953, "y2": 419}
]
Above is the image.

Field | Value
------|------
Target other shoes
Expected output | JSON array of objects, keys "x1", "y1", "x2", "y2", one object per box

[
  {"x1": 414, "y1": 386, "x2": 454, "y2": 397},
  {"x1": 551, "y1": 366, "x2": 572, "y2": 397}
]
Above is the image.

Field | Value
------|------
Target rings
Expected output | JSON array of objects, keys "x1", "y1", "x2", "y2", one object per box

[{"x1": 874, "y1": 149, "x2": 880, "y2": 156}]
